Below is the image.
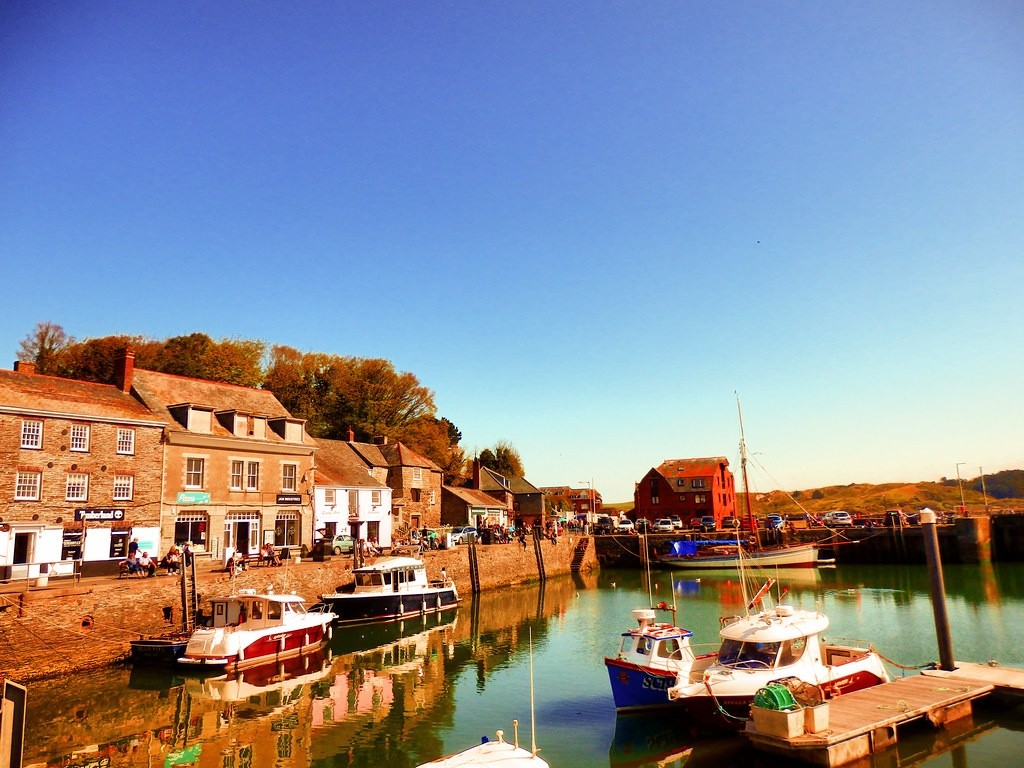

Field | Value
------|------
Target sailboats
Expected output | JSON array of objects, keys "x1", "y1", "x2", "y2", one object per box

[{"x1": 647, "y1": 389, "x2": 824, "y2": 570}]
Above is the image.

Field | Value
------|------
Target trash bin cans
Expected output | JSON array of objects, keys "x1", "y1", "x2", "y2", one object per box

[
  {"x1": 482, "y1": 528, "x2": 494, "y2": 545},
  {"x1": 887, "y1": 510, "x2": 900, "y2": 526},
  {"x1": 313, "y1": 538, "x2": 332, "y2": 562},
  {"x1": 947, "y1": 511, "x2": 956, "y2": 524},
  {"x1": 533, "y1": 525, "x2": 544, "y2": 540}
]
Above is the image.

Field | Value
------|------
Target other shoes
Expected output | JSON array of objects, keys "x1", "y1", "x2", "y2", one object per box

[
  {"x1": 167, "y1": 572, "x2": 172, "y2": 576},
  {"x1": 267, "y1": 565, "x2": 270, "y2": 568},
  {"x1": 137, "y1": 576, "x2": 142, "y2": 579},
  {"x1": 148, "y1": 574, "x2": 155, "y2": 577},
  {"x1": 142, "y1": 576, "x2": 146, "y2": 579},
  {"x1": 278, "y1": 563, "x2": 282, "y2": 566},
  {"x1": 271, "y1": 564, "x2": 274, "y2": 567},
  {"x1": 173, "y1": 572, "x2": 178, "y2": 576}
]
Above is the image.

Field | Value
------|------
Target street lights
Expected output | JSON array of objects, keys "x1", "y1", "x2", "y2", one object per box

[
  {"x1": 956, "y1": 462, "x2": 967, "y2": 517},
  {"x1": 578, "y1": 481, "x2": 594, "y2": 534}
]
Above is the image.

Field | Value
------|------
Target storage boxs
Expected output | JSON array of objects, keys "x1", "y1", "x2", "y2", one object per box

[
  {"x1": 800, "y1": 701, "x2": 829, "y2": 733},
  {"x1": 748, "y1": 703, "x2": 806, "y2": 738}
]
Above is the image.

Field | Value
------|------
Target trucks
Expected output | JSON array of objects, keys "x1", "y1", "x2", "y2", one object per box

[{"x1": 593, "y1": 517, "x2": 616, "y2": 535}]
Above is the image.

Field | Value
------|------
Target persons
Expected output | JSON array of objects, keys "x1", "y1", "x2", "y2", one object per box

[
  {"x1": 363, "y1": 537, "x2": 383, "y2": 557},
  {"x1": 546, "y1": 520, "x2": 555, "y2": 529},
  {"x1": 226, "y1": 549, "x2": 247, "y2": 578},
  {"x1": 478, "y1": 521, "x2": 513, "y2": 544},
  {"x1": 119, "y1": 552, "x2": 146, "y2": 579},
  {"x1": 260, "y1": 543, "x2": 282, "y2": 567},
  {"x1": 160, "y1": 542, "x2": 183, "y2": 575},
  {"x1": 516, "y1": 524, "x2": 535, "y2": 535},
  {"x1": 139, "y1": 551, "x2": 156, "y2": 578},
  {"x1": 422, "y1": 525, "x2": 448, "y2": 549},
  {"x1": 128, "y1": 537, "x2": 142, "y2": 558},
  {"x1": 440, "y1": 567, "x2": 447, "y2": 581}
]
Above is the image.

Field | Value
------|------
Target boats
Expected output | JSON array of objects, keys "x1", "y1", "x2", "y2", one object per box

[
  {"x1": 594, "y1": 519, "x2": 894, "y2": 734},
  {"x1": 177, "y1": 534, "x2": 345, "y2": 675},
  {"x1": 313, "y1": 544, "x2": 467, "y2": 629}
]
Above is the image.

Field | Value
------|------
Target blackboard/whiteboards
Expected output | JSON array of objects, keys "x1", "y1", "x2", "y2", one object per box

[{"x1": 280, "y1": 548, "x2": 291, "y2": 559}]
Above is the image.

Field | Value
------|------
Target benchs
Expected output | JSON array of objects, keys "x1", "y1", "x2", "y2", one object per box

[
  {"x1": 156, "y1": 561, "x2": 182, "y2": 575},
  {"x1": 119, "y1": 563, "x2": 150, "y2": 580},
  {"x1": 242, "y1": 553, "x2": 273, "y2": 567}
]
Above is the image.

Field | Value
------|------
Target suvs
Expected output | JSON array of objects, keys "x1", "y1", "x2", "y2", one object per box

[
  {"x1": 741, "y1": 517, "x2": 758, "y2": 530},
  {"x1": 700, "y1": 516, "x2": 717, "y2": 533},
  {"x1": 745, "y1": 514, "x2": 760, "y2": 528},
  {"x1": 886, "y1": 510, "x2": 907, "y2": 526}
]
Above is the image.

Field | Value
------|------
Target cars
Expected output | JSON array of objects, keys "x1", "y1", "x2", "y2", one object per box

[
  {"x1": 635, "y1": 517, "x2": 683, "y2": 533},
  {"x1": 721, "y1": 516, "x2": 741, "y2": 528},
  {"x1": 824, "y1": 510, "x2": 853, "y2": 528},
  {"x1": 317, "y1": 527, "x2": 357, "y2": 556},
  {"x1": 690, "y1": 518, "x2": 701, "y2": 528},
  {"x1": 906, "y1": 513, "x2": 942, "y2": 526},
  {"x1": 764, "y1": 513, "x2": 786, "y2": 531},
  {"x1": 450, "y1": 525, "x2": 478, "y2": 545},
  {"x1": 616, "y1": 520, "x2": 635, "y2": 534}
]
